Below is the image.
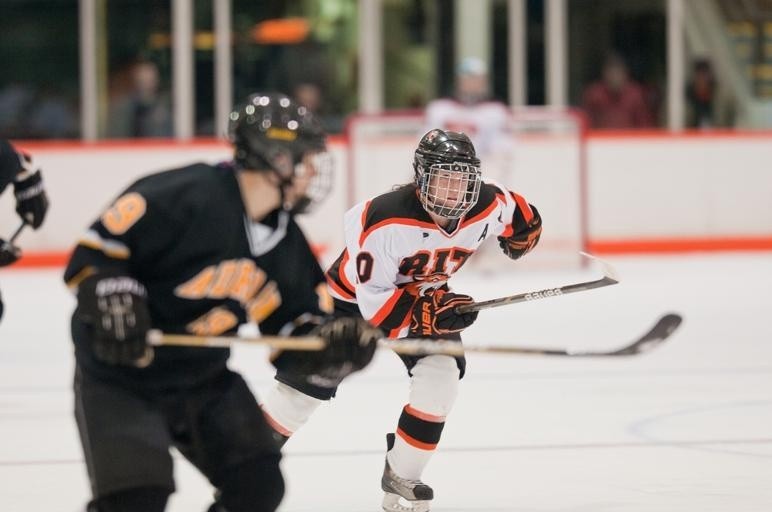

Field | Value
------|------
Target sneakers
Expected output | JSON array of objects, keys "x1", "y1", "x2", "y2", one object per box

[{"x1": 381, "y1": 460, "x2": 434, "y2": 500}]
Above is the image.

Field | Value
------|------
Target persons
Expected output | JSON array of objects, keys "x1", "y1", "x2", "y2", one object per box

[
  {"x1": 291, "y1": 79, "x2": 339, "y2": 131},
  {"x1": 578, "y1": 55, "x2": 654, "y2": 128},
  {"x1": 420, "y1": 55, "x2": 514, "y2": 153},
  {"x1": 0, "y1": 135, "x2": 51, "y2": 318},
  {"x1": 64, "y1": 91, "x2": 383, "y2": 511},
  {"x1": 94, "y1": 58, "x2": 192, "y2": 139},
  {"x1": 656, "y1": 56, "x2": 747, "y2": 129},
  {"x1": 213, "y1": 128, "x2": 543, "y2": 510}
]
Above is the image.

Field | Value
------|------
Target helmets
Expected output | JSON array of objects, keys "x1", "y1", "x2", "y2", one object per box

[
  {"x1": 413, "y1": 129, "x2": 483, "y2": 220},
  {"x1": 226, "y1": 90, "x2": 336, "y2": 213}
]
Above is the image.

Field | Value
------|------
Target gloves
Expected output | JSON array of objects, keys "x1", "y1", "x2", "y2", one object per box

[
  {"x1": 498, "y1": 203, "x2": 542, "y2": 259},
  {"x1": 71, "y1": 267, "x2": 153, "y2": 368},
  {"x1": 13, "y1": 162, "x2": 49, "y2": 228},
  {"x1": 315, "y1": 315, "x2": 384, "y2": 380},
  {"x1": 407, "y1": 287, "x2": 479, "y2": 338}
]
